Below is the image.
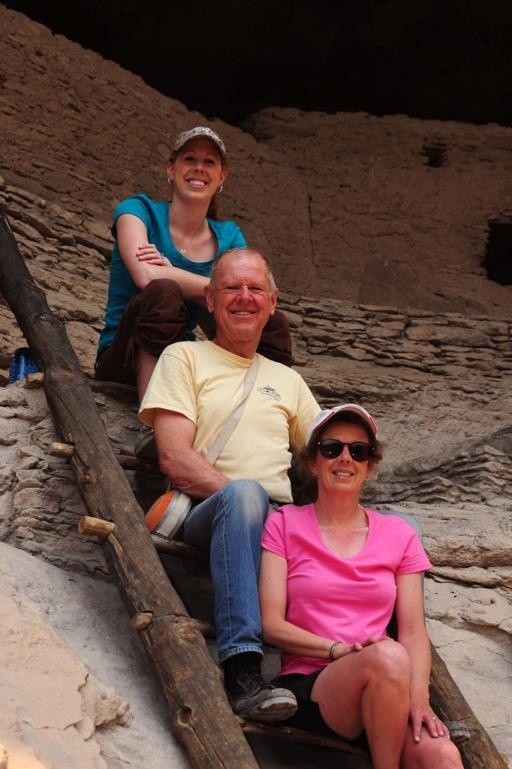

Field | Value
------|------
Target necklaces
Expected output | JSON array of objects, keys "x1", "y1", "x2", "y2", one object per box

[{"x1": 169, "y1": 213, "x2": 206, "y2": 254}]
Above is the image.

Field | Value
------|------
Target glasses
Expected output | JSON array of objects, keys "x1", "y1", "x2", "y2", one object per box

[{"x1": 314, "y1": 439, "x2": 376, "y2": 461}]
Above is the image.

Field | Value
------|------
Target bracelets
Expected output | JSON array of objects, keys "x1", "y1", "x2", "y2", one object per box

[{"x1": 328, "y1": 638, "x2": 345, "y2": 659}]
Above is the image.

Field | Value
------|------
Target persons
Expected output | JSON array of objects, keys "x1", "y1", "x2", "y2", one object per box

[
  {"x1": 91, "y1": 123, "x2": 293, "y2": 410},
  {"x1": 261, "y1": 399, "x2": 465, "y2": 768},
  {"x1": 135, "y1": 241, "x2": 320, "y2": 726}
]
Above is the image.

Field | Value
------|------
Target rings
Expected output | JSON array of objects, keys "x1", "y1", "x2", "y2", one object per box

[{"x1": 160, "y1": 252, "x2": 165, "y2": 259}]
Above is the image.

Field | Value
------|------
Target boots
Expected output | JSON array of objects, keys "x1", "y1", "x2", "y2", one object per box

[
  {"x1": 440, "y1": 719, "x2": 473, "y2": 744},
  {"x1": 228, "y1": 663, "x2": 297, "y2": 722}
]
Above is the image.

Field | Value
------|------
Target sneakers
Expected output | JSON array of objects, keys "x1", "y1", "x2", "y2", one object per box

[{"x1": 135, "y1": 426, "x2": 155, "y2": 455}]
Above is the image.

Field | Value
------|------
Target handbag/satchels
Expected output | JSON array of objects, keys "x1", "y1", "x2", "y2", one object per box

[{"x1": 146, "y1": 490, "x2": 192, "y2": 539}]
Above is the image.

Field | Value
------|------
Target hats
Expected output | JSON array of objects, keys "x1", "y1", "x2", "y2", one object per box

[
  {"x1": 307, "y1": 403, "x2": 376, "y2": 447},
  {"x1": 174, "y1": 126, "x2": 227, "y2": 160}
]
